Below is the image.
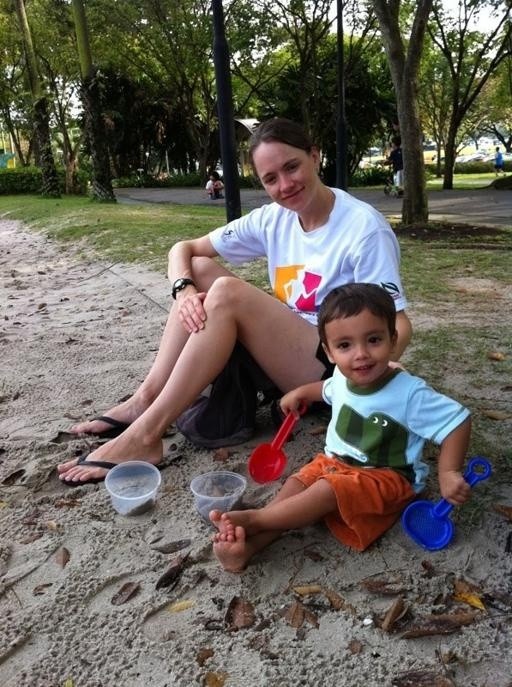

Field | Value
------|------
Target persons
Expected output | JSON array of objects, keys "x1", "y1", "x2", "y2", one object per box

[
  {"x1": 490, "y1": 147, "x2": 506, "y2": 178},
  {"x1": 208, "y1": 283, "x2": 473, "y2": 573},
  {"x1": 206, "y1": 171, "x2": 224, "y2": 199},
  {"x1": 0, "y1": 147, "x2": 16, "y2": 170},
  {"x1": 159, "y1": 167, "x2": 168, "y2": 179},
  {"x1": 56, "y1": 118, "x2": 412, "y2": 486},
  {"x1": 391, "y1": 118, "x2": 401, "y2": 137},
  {"x1": 69, "y1": 156, "x2": 76, "y2": 167},
  {"x1": 431, "y1": 155, "x2": 436, "y2": 161},
  {"x1": 383, "y1": 136, "x2": 405, "y2": 194}
]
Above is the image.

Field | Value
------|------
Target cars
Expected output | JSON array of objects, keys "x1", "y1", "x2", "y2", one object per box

[{"x1": 438, "y1": 152, "x2": 512, "y2": 162}]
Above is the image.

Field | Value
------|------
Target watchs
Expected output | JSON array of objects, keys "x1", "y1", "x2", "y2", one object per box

[{"x1": 172, "y1": 278, "x2": 196, "y2": 300}]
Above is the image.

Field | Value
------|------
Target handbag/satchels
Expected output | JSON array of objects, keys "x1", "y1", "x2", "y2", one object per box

[{"x1": 178, "y1": 383, "x2": 278, "y2": 448}]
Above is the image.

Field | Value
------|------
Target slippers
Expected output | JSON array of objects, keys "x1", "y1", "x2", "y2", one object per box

[
  {"x1": 81, "y1": 416, "x2": 177, "y2": 440},
  {"x1": 61, "y1": 452, "x2": 169, "y2": 486}
]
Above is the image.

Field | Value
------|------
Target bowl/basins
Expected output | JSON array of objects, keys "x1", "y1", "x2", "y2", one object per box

[
  {"x1": 188, "y1": 470, "x2": 249, "y2": 526},
  {"x1": 105, "y1": 460, "x2": 163, "y2": 517}
]
